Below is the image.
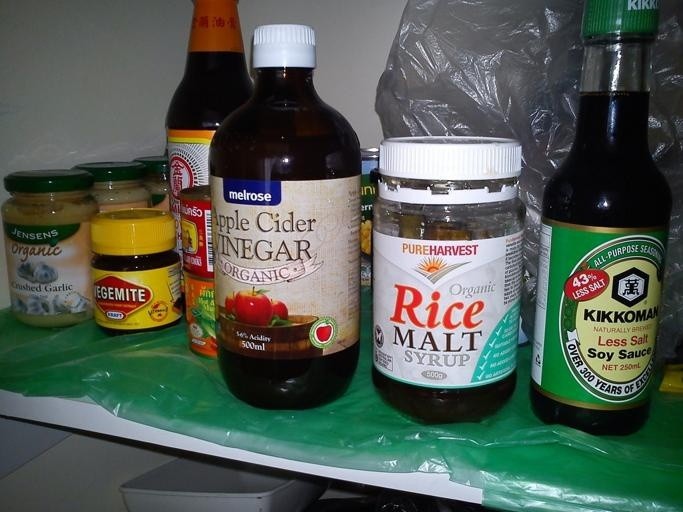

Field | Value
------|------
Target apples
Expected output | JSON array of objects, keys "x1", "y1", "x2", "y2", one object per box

[
  {"x1": 317, "y1": 325, "x2": 331, "y2": 342},
  {"x1": 224, "y1": 284, "x2": 288, "y2": 326}
]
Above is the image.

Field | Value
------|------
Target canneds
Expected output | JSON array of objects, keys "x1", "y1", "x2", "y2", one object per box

[
  {"x1": 74, "y1": 161, "x2": 152, "y2": 211},
  {"x1": 185, "y1": 275, "x2": 216, "y2": 358},
  {"x1": 132, "y1": 155, "x2": 170, "y2": 207},
  {"x1": 180, "y1": 185, "x2": 215, "y2": 279},
  {"x1": 90, "y1": 210, "x2": 184, "y2": 332},
  {"x1": 360, "y1": 147, "x2": 379, "y2": 285},
  {"x1": 3, "y1": 168, "x2": 98, "y2": 328},
  {"x1": 374, "y1": 137, "x2": 523, "y2": 421}
]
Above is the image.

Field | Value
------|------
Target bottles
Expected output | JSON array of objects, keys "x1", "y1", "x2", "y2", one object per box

[
  {"x1": 175, "y1": 185, "x2": 219, "y2": 280},
  {"x1": 169, "y1": 0, "x2": 256, "y2": 289},
  {"x1": 208, "y1": 23, "x2": 361, "y2": 411},
  {"x1": 528, "y1": 0, "x2": 671, "y2": 437},
  {"x1": 178, "y1": 271, "x2": 216, "y2": 358}
]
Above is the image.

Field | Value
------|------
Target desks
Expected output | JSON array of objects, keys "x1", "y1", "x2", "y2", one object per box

[{"x1": 0, "y1": 280, "x2": 682, "y2": 511}]
